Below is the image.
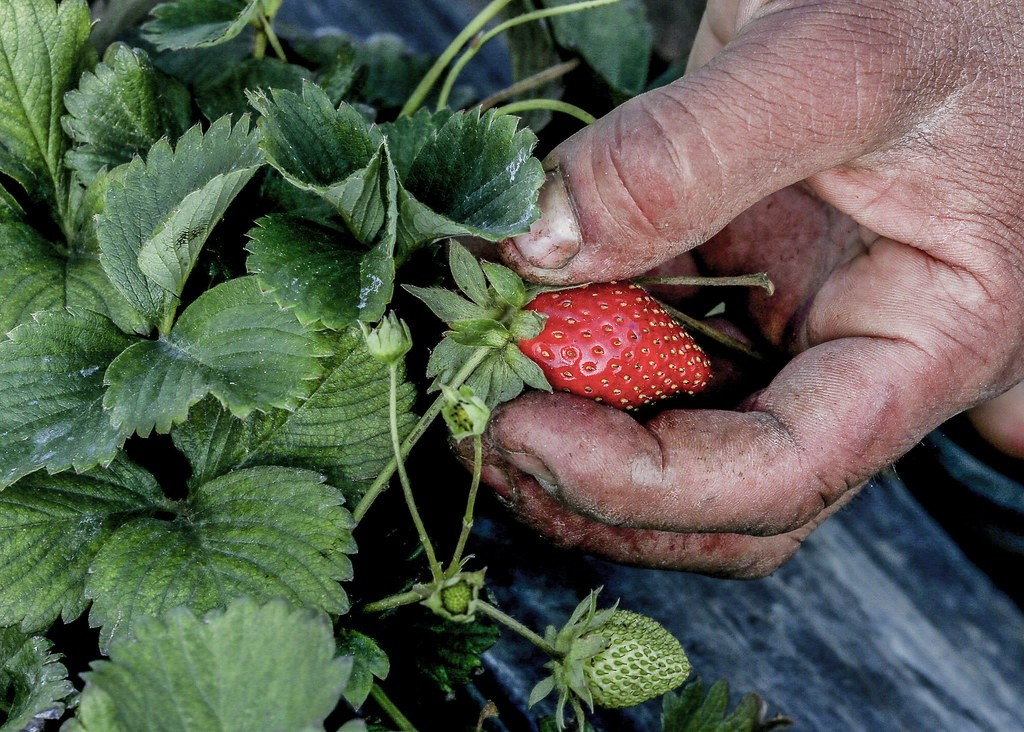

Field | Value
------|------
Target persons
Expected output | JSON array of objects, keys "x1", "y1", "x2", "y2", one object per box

[{"x1": 445, "y1": 0, "x2": 1024, "y2": 581}]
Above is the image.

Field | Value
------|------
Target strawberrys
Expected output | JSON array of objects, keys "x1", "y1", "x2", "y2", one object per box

[
  {"x1": 508, "y1": 277, "x2": 719, "y2": 413},
  {"x1": 571, "y1": 608, "x2": 693, "y2": 708}
]
